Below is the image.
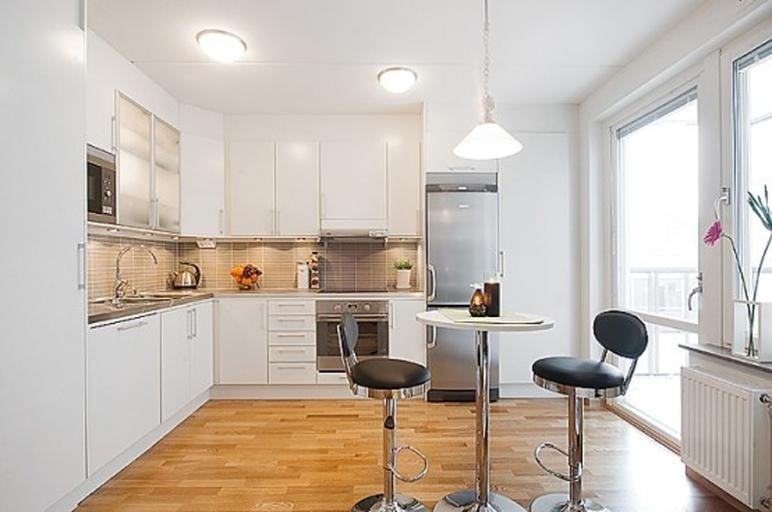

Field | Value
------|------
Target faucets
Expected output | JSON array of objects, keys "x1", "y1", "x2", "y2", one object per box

[{"x1": 115, "y1": 245, "x2": 157, "y2": 297}]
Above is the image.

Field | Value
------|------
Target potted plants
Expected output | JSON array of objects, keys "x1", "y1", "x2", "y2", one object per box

[{"x1": 393, "y1": 258, "x2": 414, "y2": 289}]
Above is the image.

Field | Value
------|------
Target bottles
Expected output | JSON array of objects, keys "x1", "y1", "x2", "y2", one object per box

[
  {"x1": 297, "y1": 261, "x2": 310, "y2": 289},
  {"x1": 469, "y1": 289, "x2": 488, "y2": 316},
  {"x1": 310, "y1": 250, "x2": 322, "y2": 290}
]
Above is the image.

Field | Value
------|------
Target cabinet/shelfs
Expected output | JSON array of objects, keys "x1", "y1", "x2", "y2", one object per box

[
  {"x1": 225, "y1": 114, "x2": 321, "y2": 243},
  {"x1": 387, "y1": 113, "x2": 421, "y2": 238},
  {"x1": 161, "y1": 298, "x2": 214, "y2": 439},
  {"x1": 214, "y1": 295, "x2": 269, "y2": 400},
  {"x1": 269, "y1": 293, "x2": 318, "y2": 399},
  {"x1": 114, "y1": 89, "x2": 183, "y2": 234},
  {"x1": 1, "y1": 0, "x2": 86, "y2": 512},
  {"x1": 320, "y1": 113, "x2": 387, "y2": 236},
  {"x1": 388, "y1": 297, "x2": 428, "y2": 369},
  {"x1": 180, "y1": 102, "x2": 226, "y2": 237}
]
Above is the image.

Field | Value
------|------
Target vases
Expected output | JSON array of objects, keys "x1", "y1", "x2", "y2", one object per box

[{"x1": 732, "y1": 300, "x2": 772, "y2": 364}]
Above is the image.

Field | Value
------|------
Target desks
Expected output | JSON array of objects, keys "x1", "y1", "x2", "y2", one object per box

[{"x1": 416, "y1": 309, "x2": 554, "y2": 512}]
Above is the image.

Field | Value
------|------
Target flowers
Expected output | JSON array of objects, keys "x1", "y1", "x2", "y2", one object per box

[{"x1": 702, "y1": 185, "x2": 772, "y2": 358}]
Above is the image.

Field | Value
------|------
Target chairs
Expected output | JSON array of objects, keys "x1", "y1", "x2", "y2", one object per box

[
  {"x1": 531, "y1": 310, "x2": 649, "y2": 512},
  {"x1": 335, "y1": 311, "x2": 432, "y2": 512}
]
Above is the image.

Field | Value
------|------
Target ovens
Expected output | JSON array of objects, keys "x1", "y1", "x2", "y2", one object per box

[{"x1": 315, "y1": 314, "x2": 390, "y2": 373}]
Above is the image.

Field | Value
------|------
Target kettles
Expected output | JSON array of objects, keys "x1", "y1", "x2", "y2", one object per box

[{"x1": 170, "y1": 260, "x2": 202, "y2": 290}]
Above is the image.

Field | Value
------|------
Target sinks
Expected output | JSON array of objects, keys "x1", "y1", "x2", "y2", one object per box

[
  {"x1": 139, "y1": 295, "x2": 188, "y2": 299},
  {"x1": 94, "y1": 299, "x2": 165, "y2": 304}
]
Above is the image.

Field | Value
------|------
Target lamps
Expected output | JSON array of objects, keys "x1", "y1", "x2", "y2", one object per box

[
  {"x1": 376, "y1": 66, "x2": 417, "y2": 94},
  {"x1": 196, "y1": 29, "x2": 246, "y2": 65},
  {"x1": 453, "y1": 0, "x2": 524, "y2": 161}
]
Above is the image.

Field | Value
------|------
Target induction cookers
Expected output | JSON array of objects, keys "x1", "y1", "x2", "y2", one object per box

[{"x1": 317, "y1": 286, "x2": 388, "y2": 315}]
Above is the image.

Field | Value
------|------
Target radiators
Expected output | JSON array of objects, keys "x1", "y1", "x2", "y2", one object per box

[{"x1": 681, "y1": 365, "x2": 772, "y2": 512}]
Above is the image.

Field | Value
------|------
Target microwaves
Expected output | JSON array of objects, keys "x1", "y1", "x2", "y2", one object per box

[{"x1": 86, "y1": 144, "x2": 118, "y2": 225}]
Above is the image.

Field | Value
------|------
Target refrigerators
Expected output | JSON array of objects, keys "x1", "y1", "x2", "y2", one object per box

[{"x1": 425, "y1": 173, "x2": 502, "y2": 402}]
